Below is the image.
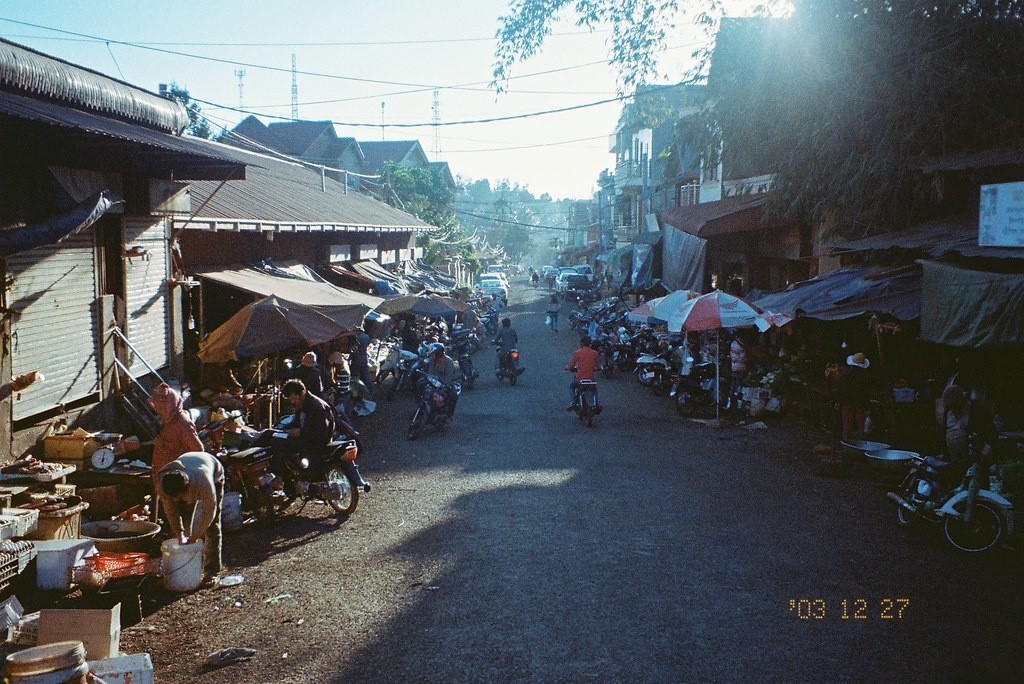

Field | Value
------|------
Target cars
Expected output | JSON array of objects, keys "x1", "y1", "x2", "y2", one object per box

[
  {"x1": 475, "y1": 265, "x2": 509, "y2": 306},
  {"x1": 541, "y1": 266, "x2": 559, "y2": 286}
]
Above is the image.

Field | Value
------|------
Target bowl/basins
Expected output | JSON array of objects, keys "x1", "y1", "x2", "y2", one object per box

[
  {"x1": 840, "y1": 439, "x2": 891, "y2": 462},
  {"x1": 81, "y1": 521, "x2": 161, "y2": 554},
  {"x1": 864, "y1": 450, "x2": 921, "y2": 475}
]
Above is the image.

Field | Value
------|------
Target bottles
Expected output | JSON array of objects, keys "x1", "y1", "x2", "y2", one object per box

[{"x1": 864, "y1": 416, "x2": 872, "y2": 433}]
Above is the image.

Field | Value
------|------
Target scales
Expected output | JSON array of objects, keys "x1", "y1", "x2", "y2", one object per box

[{"x1": 90, "y1": 433, "x2": 123, "y2": 474}]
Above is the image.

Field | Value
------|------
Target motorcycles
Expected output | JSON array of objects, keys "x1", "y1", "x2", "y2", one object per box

[
  {"x1": 886, "y1": 433, "x2": 1013, "y2": 553},
  {"x1": 317, "y1": 383, "x2": 364, "y2": 453},
  {"x1": 406, "y1": 369, "x2": 462, "y2": 440},
  {"x1": 566, "y1": 296, "x2": 725, "y2": 416},
  {"x1": 491, "y1": 339, "x2": 525, "y2": 386},
  {"x1": 272, "y1": 409, "x2": 371, "y2": 515},
  {"x1": 375, "y1": 305, "x2": 498, "y2": 394},
  {"x1": 565, "y1": 366, "x2": 603, "y2": 427},
  {"x1": 197, "y1": 411, "x2": 276, "y2": 524}
]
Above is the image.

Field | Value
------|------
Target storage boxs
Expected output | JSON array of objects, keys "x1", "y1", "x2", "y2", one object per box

[
  {"x1": 45, "y1": 429, "x2": 105, "y2": 471},
  {"x1": 82, "y1": 551, "x2": 149, "y2": 582},
  {"x1": 26, "y1": 540, "x2": 95, "y2": 591},
  {"x1": 0, "y1": 595, "x2": 121, "y2": 660},
  {"x1": 0, "y1": 508, "x2": 39, "y2": 595},
  {"x1": 12, "y1": 483, "x2": 76, "y2": 508},
  {"x1": 88, "y1": 654, "x2": 154, "y2": 684}
]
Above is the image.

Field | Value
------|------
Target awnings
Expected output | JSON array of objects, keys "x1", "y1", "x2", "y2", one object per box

[
  {"x1": 756, "y1": 255, "x2": 924, "y2": 321},
  {"x1": 559, "y1": 193, "x2": 796, "y2": 259},
  {"x1": 330, "y1": 259, "x2": 458, "y2": 299},
  {"x1": 194, "y1": 257, "x2": 385, "y2": 329}
]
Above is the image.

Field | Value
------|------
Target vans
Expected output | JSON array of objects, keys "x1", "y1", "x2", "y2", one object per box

[{"x1": 572, "y1": 264, "x2": 593, "y2": 285}]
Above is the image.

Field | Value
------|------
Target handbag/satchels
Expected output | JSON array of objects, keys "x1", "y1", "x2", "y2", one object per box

[{"x1": 545, "y1": 315, "x2": 552, "y2": 325}]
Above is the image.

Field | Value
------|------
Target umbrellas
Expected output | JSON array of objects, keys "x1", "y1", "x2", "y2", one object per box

[
  {"x1": 630, "y1": 289, "x2": 701, "y2": 376},
  {"x1": 199, "y1": 295, "x2": 371, "y2": 380},
  {"x1": 379, "y1": 293, "x2": 471, "y2": 339},
  {"x1": 669, "y1": 288, "x2": 763, "y2": 416}
]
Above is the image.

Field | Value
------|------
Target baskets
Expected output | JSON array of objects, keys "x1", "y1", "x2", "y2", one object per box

[
  {"x1": 71, "y1": 552, "x2": 149, "y2": 583},
  {"x1": 31, "y1": 483, "x2": 76, "y2": 499},
  {"x1": 1, "y1": 508, "x2": 40, "y2": 540},
  {"x1": 7, "y1": 611, "x2": 41, "y2": 646},
  {"x1": 0, "y1": 595, "x2": 25, "y2": 631}
]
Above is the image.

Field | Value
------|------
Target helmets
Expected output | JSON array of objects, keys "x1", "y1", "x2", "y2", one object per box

[{"x1": 428, "y1": 342, "x2": 444, "y2": 361}]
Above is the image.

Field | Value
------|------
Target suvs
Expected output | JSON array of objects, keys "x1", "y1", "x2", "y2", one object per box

[{"x1": 556, "y1": 267, "x2": 592, "y2": 301}]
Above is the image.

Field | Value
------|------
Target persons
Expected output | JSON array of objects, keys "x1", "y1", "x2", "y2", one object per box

[
  {"x1": 730, "y1": 333, "x2": 753, "y2": 392},
  {"x1": 282, "y1": 331, "x2": 380, "y2": 497},
  {"x1": 149, "y1": 385, "x2": 205, "y2": 536},
  {"x1": 427, "y1": 343, "x2": 457, "y2": 420},
  {"x1": 547, "y1": 295, "x2": 561, "y2": 333},
  {"x1": 942, "y1": 385, "x2": 993, "y2": 506},
  {"x1": 565, "y1": 336, "x2": 603, "y2": 413},
  {"x1": 836, "y1": 353, "x2": 870, "y2": 441},
  {"x1": 495, "y1": 318, "x2": 518, "y2": 373},
  {"x1": 490, "y1": 294, "x2": 504, "y2": 334},
  {"x1": 158, "y1": 452, "x2": 225, "y2": 586},
  {"x1": 528, "y1": 263, "x2": 539, "y2": 287}
]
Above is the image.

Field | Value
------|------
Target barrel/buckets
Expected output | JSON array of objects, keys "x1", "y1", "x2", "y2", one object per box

[
  {"x1": 4, "y1": 641, "x2": 89, "y2": 684},
  {"x1": 749, "y1": 397, "x2": 768, "y2": 419},
  {"x1": 221, "y1": 491, "x2": 243, "y2": 533},
  {"x1": 156, "y1": 537, "x2": 204, "y2": 591}
]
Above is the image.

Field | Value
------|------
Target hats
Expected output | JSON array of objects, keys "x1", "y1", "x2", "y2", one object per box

[
  {"x1": 329, "y1": 352, "x2": 343, "y2": 364},
  {"x1": 302, "y1": 351, "x2": 317, "y2": 366},
  {"x1": 847, "y1": 353, "x2": 870, "y2": 369}
]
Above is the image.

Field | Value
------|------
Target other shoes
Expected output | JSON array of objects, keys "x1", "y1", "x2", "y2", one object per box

[
  {"x1": 496, "y1": 369, "x2": 500, "y2": 373},
  {"x1": 199, "y1": 575, "x2": 220, "y2": 588},
  {"x1": 566, "y1": 404, "x2": 577, "y2": 412},
  {"x1": 554, "y1": 329, "x2": 558, "y2": 333},
  {"x1": 596, "y1": 404, "x2": 602, "y2": 410}
]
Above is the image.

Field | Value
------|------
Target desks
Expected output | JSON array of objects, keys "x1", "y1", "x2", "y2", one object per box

[{"x1": 75, "y1": 467, "x2": 161, "y2": 524}]
